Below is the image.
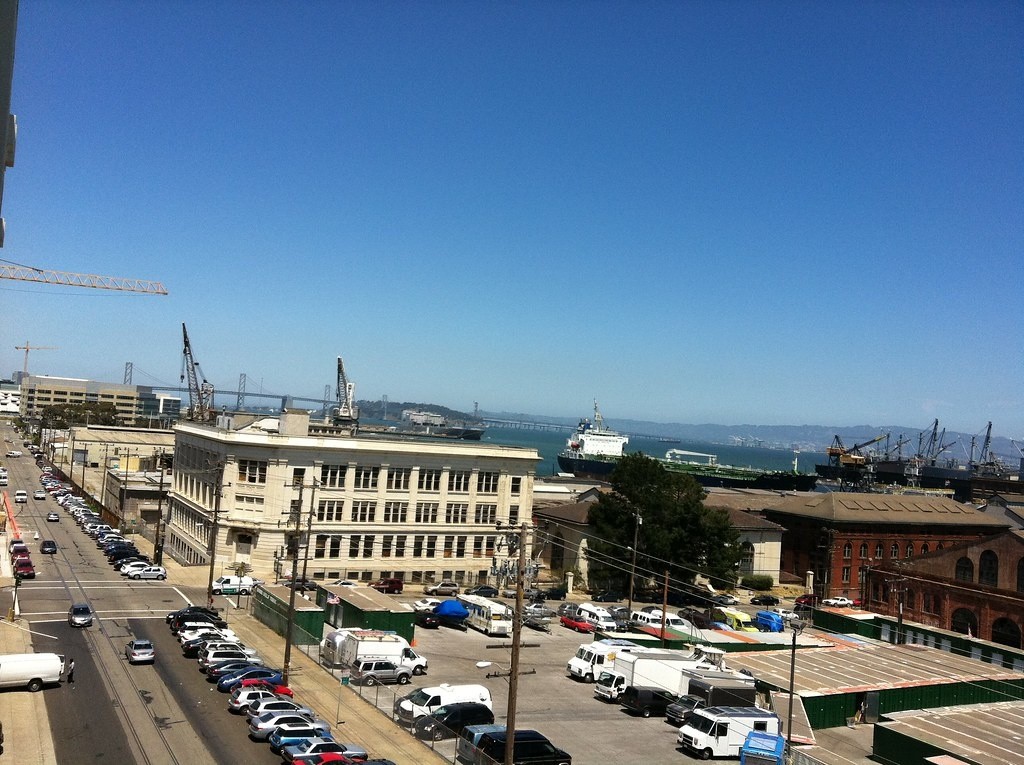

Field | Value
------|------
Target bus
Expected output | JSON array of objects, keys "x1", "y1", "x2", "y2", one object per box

[{"x1": 456, "y1": 593, "x2": 512, "y2": 636}]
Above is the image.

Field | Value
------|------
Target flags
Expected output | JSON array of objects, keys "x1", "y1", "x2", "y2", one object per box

[{"x1": 325, "y1": 591, "x2": 340, "y2": 605}]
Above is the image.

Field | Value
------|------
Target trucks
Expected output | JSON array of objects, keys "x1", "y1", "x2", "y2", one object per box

[
  {"x1": 665, "y1": 676, "x2": 757, "y2": 724},
  {"x1": 676, "y1": 704, "x2": 780, "y2": 759},
  {"x1": 567, "y1": 637, "x2": 706, "y2": 702},
  {"x1": 319, "y1": 624, "x2": 429, "y2": 676}
]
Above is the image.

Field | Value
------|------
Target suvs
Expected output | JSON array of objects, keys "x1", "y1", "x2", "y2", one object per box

[
  {"x1": 619, "y1": 684, "x2": 683, "y2": 719},
  {"x1": 352, "y1": 657, "x2": 413, "y2": 685}
]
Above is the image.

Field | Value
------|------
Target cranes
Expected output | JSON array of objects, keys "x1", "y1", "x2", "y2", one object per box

[
  {"x1": 181, "y1": 324, "x2": 214, "y2": 424},
  {"x1": 335, "y1": 355, "x2": 360, "y2": 426},
  {"x1": 15, "y1": 342, "x2": 59, "y2": 373}
]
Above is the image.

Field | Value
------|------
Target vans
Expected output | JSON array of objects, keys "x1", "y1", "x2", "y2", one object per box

[
  {"x1": 458, "y1": 725, "x2": 572, "y2": 765},
  {"x1": 397, "y1": 684, "x2": 492, "y2": 725},
  {"x1": 0, "y1": 653, "x2": 66, "y2": 691},
  {"x1": 213, "y1": 575, "x2": 252, "y2": 596}
]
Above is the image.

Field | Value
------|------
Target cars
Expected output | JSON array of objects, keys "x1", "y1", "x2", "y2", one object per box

[
  {"x1": 4, "y1": 419, "x2": 165, "y2": 581},
  {"x1": 67, "y1": 604, "x2": 95, "y2": 629},
  {"x1": 278, "y1": 577, "x2": 863, "y2": 631},
  {"x1": 166, "y1": 605, "x2": 399, "y2": 765},
  {"x1": 124, "y1": 639, "x2": 155, "y2": 664},
  {"x1": 394, "y1": 684, "x2": 494, "y2": 741}
]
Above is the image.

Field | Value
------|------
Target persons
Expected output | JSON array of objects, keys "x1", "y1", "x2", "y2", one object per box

[
  {"x1": 188, "y1": 604, "x2": 191, "y2": 608},
  {"x1": 67, "y1": 659, "x2": 75, "y2": 684}
]
